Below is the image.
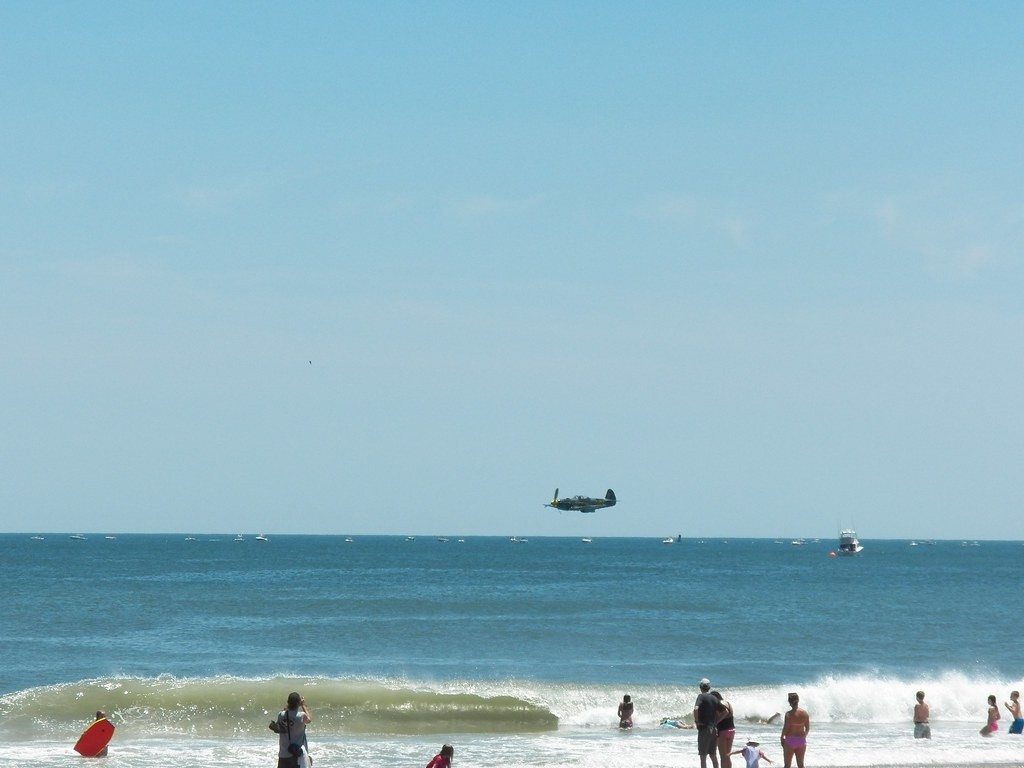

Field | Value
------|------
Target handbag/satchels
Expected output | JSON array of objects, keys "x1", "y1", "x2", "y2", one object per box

[{"x1": 288, "y1": 743, "x2": 303, "y2": 757}]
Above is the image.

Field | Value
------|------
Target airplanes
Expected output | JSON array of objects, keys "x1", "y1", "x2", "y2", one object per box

[{"x1": 543, "y1": 486, "x2": 618, "y2": 514}]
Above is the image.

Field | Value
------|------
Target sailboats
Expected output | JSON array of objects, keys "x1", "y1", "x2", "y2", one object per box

[{"x1": 676, "y1": 535, "x2": 682, "y2": 543}]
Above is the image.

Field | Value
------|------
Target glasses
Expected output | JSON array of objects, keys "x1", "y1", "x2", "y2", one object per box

[{"x1": 789, "y1": 693, "x2": 797, "y2": 698}]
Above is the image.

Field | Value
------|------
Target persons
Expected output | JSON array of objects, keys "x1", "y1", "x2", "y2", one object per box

[
  {"x1": 727, "y1": 737, "x2": 773, "y2": 768},
  {"x1": 694, "y1": 678, "x2": 735, "y2": 768},
  {"x1": 987, "y1": 695, "x2": 1001, "y2": 732},
  {"x1": 426, "y1": 744, "x2": 454, "y2": 768},
  {"x1": 1004, "y1": 691, "x2": 1024, "y2": 734},
  {"x1": 913, "y1": 691, "x2": 931, "y2": 738},
  {"x1": 781, "y1": 693, "x2": 809, "y2": 768},
  {"x1": 618, "y1": 695, "x2": 633, "y2": 728},
  {"x1": 276, "y1": 692, "x2": 313, "y2": 768},
  {"x1": 95, "y1": 710, "x2": 108, "y2": 757}
]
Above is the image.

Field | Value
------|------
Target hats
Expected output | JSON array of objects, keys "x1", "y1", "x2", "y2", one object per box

[
  {"x1": 660, "y1": 717, "x2": 669, "y2": 723},
  {"x1": 699, "y1": 678, "x2": 710, "y2": 685},
  {"x1": 746, "y1": 738, "x2": 759, "y2": 747}
]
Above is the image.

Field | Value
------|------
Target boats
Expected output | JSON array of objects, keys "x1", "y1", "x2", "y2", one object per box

[
  {"x1": 792, "y1": 538, "x2": 808, "y2": 546},
  {"x1": 438, "y1": 537, "x2": 450, "y2": 542},
  {"x1": 775, "y1": 542, "x2": 783, "y2": 544},
  {"x1": 909, "y1": 539, "x2": 982, "y2": 547},
  {"x1": 459, "y1": 539, "x2": 465, "y2": 543},
  {"x1": 255, "y1": 535, "x2": 268, "y2": 542},
  {"x1": 509, "y1": 536, "x2": 518, "y2": 542},
  {"x1": 233, "y1": 536, "x2": 245, "y2": 541},
  {"x1": 105, "y1": 536, "x2": 115, "y2": 539},
  {"x1": 664, "y1": 537, "x2": 673, "y2": 543},
  {"x1": 69, "y1": 533, "x2": 87, "y2": 540},
  {"x1": 345, "y1": 538, "x2": 353, "y2": 543},
  {"x1": 520, "y1": 538, "x2": 529, "y2": 543},
  {"x1": 406, "y1": 537, "x2": 415, "y2": 542},
  {"x1": 834, "y1": 530, "x2": 866, "y2": 557},
  {"x1": 582, "y1": 536, "x2": 593, "y2": 544},
  {"x1": 32, "y1": 536, "x2": 47, "y2": 541},
  {"x1": 186, "y1": 537, "x2": 200, "y2": 543}
]
